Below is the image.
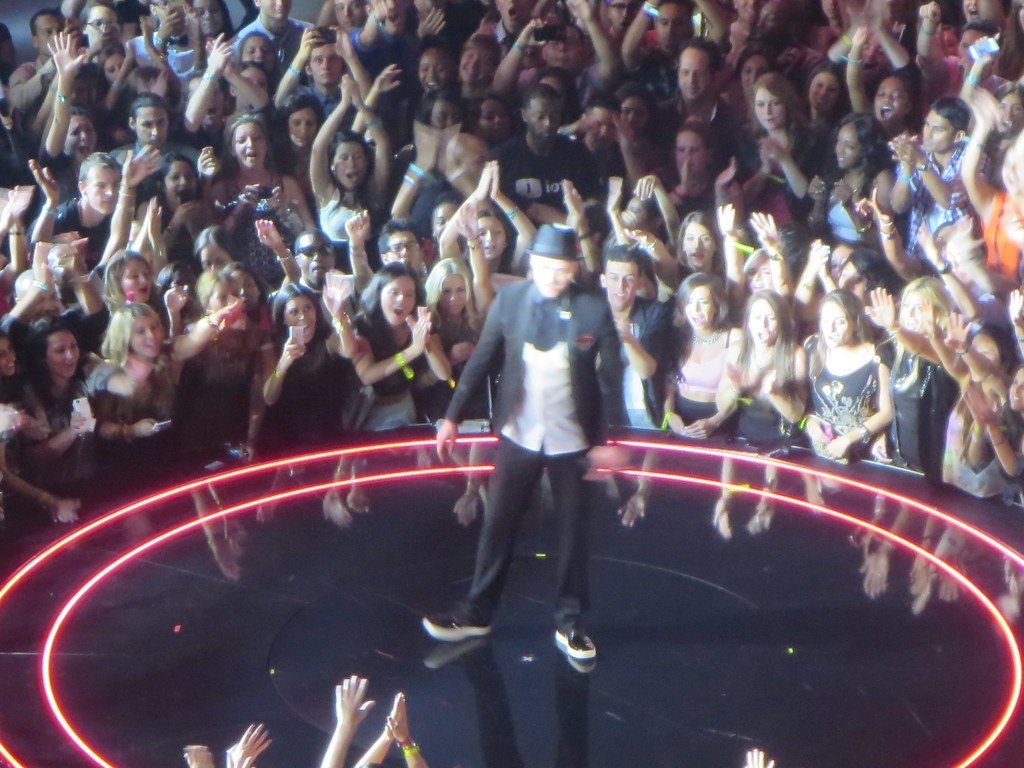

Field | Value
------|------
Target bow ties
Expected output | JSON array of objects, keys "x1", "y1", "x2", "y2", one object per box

[{"x1": 167, "y1": 36, "x2": 188, "y2": 45}]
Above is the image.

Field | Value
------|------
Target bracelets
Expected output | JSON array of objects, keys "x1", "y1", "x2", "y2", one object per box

[
  {"x1": 271, "y1": 371, "x2": 284, "y2": 385},
  {"x1": 937, "y1": 262, "x2": 951, "y2": 274},
  {"x1": 958, "y1": 346, "x2": 969, "y2": 355},
  {"x1": 506, "y1": 208, "x2": 518, "y2": 219},
  {"x1": 397, "y1": 351, "x2": 414, "y2": 379},
  {"x1": 578, "y1": 229, "x2": 593, "y2": 241},
  {"x1": 662, "y1": 412, "x2": 674, "y2": 430},
  {"x1": 878, "y1": 223, "x2": 897, "y2": 239},
  {"x1": 919, "y1": 160, "x2": 932, "y2": 171},
  {"x1": 171, "y1": 32, "x2": 188, "y2": 47},
  {"x1": 470, "y1": 242, "x2": 483, "y2": 251},
  {"x1": 366, "y1": 118, "x2": 381, "y2": 129},
  {"x1": 181, "y1": 722, "x2": 271, "y2": 768},
  {"x1": 395, "y1": 737, "x2": 419, "y2": 758}
]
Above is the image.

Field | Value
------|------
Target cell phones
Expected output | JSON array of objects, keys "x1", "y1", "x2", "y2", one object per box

[
  {"x1": 969, "y1": 37, "x2": 1001, "y2": 60},
  {"x1": 73, "y1": 397, "x2": 94, "y2": 433},
  {"x1": 821, "y1": 425, "x2": 835, "y2": 441},
  {"x1": 153, "y1": 419, "x2": 172, "y2": 430},
  {"x1": 180, "y1": 188, "x2": 194, "y2": 205},
  {"x1": 535, "y1": 24, "x2": 567, "y2": 40},
  {"x1": 290, "y1": 326, "x2": 305, "y2": 357}
]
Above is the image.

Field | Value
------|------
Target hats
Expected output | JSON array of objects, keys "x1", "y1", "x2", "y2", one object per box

[{"x1": 523, "y1": 223, "x2": 585, "y2": 261}]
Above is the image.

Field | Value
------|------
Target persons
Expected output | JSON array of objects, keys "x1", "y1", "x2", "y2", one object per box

[
  {"x1": 321, "y1": 676, "x2": 376, "y2": 768},
  {"x1": 420, "y1": 224, "x2": 625, "y2": 659},
  {"x1": 353, "y1": 692, "x2": 427, "y2": 768},
  {"x1": 0, "y1": 0, "x2": 1024, "y2": 523}
]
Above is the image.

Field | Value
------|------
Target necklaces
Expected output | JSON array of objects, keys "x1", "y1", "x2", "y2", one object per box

[{"x1": 691, "y1": 328, "x2": 723, "y2": 346}]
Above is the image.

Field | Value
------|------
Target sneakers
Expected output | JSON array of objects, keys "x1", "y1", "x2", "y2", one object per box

[
  {"x1": 555, "y1": 624, "x2": 596, "y2": 658},
  {"x1": 423, "y1": 612, "x2": 491, "y2": 641}
]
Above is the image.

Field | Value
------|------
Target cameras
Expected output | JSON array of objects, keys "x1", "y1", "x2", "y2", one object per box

[{"x1": 256, "y1": 186, "x2": 274, "y2": 198}]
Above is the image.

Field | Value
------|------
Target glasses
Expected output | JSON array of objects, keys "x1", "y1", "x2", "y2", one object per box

[
  {"x1": 378, "y1": 241, "x2": 420, "y2": 253},
  {"x1": 297, "y1": 243, "x2": 335, "y2": 257},
  {"x1": 82, "y1": 18, "x2": 113, "y2": 28},
  {"x1": 609, "y1": 2, "x2": 640, "y2": 9}
]
